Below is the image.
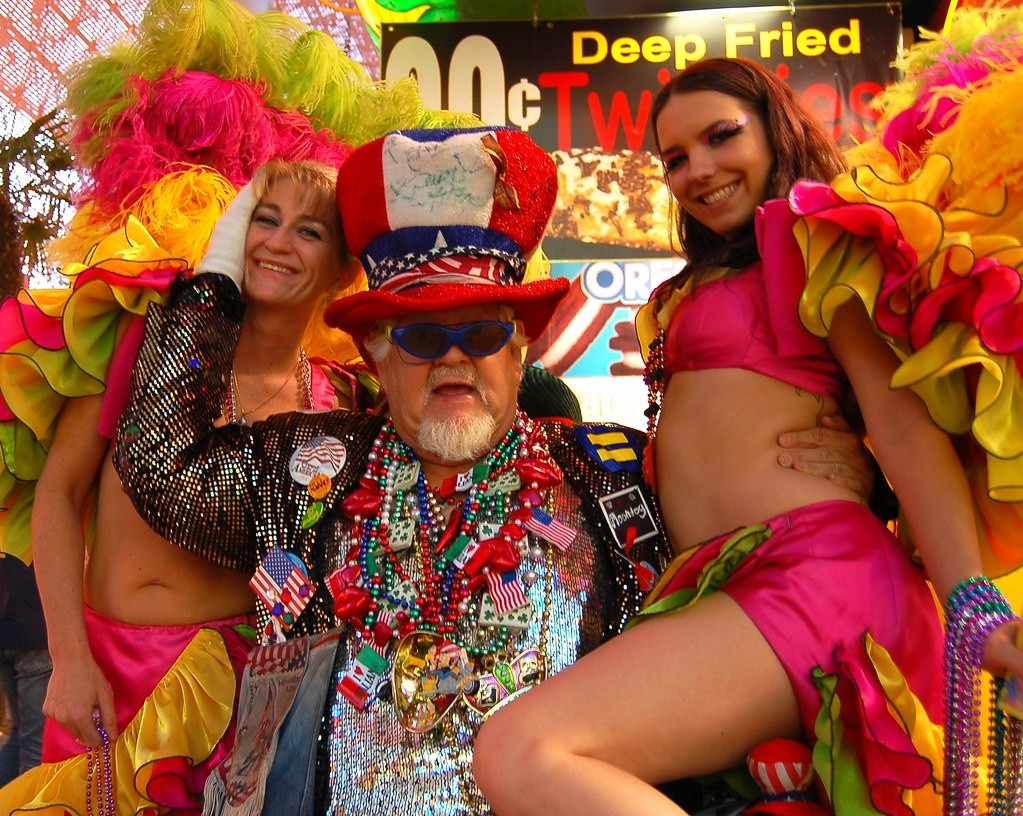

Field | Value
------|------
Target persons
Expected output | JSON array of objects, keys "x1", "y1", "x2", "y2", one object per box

[
  {"x1": 113, "y1": 167, "x2": 900, "y2": 816},
  {"x1": 34, "y1": 158, "x2": 362, "y2": 816},
  {"x1": 472, "y1": 58, "x2": 1023, "y2": 816}
]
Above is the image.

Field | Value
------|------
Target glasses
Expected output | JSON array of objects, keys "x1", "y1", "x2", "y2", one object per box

[{"x1": 385, "y1": 319, "x2": 517, "y2": 359}]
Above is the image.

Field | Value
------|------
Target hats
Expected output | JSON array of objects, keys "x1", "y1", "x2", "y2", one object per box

[{"x1": 324, "y1": 126, "x2": 571, "y2": 375}]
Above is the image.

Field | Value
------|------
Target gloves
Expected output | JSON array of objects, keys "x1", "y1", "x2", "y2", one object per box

[{"x1": 195, "y1": 172, "x2": 268, "y2": 296}]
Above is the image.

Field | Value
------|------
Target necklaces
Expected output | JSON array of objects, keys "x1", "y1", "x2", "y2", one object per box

[
  {"x1": 941, "y1": 576, "x2": 1023, "y2": 816},
  {"x1": 643, "y1": 326, "x2": 673, "y2": 561},
  {"x1": 326, "y1": 404, "x2": 565, "y2": 734},
  {"x1": 85, "y1": 726, "x2": 115, "y2": 816},
  {"x1": 221, "y1": 344, "x2": 314, "y2": 423}
]
